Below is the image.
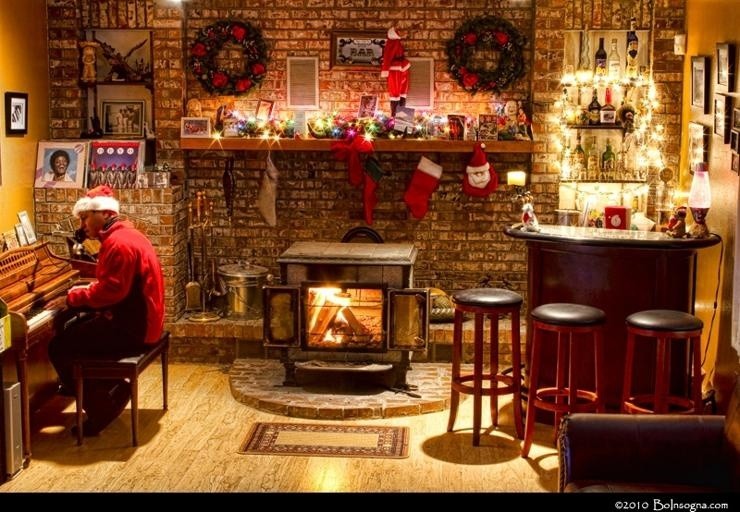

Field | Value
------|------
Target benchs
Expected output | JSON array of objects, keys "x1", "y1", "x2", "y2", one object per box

[{"x1": 71, "y1": 331, "x2": 171, "y2": 448}]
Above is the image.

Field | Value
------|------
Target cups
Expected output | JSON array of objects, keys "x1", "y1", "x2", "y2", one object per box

[
  {"x1": 566, "y1": 210, "x2": 582, "y2": 226},
  {"x1": 554, "y1": 209, "x2": 567, "y2": 225}
]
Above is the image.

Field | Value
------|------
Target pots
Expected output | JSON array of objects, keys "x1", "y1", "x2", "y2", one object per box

[{"x1": 217, "y1": 259, "x2": 274, "y2": 320}]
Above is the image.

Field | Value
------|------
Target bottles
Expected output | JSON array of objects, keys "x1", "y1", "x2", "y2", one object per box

[
  {"x1": 602, "y1": 138, "x2": 616, "y2": 170},
  {"x1": 567, "y1": 97, "x2": 576, "y2": 125},
  {"x1": 576, "y1": 105, "x2": 590, "y2": 125},
  {"x1": 571, "y1": 135, "x2": 586, "y2": 168},
  {"x1": 615, "y1": 142, "x2": 628, "y2": 171},
  {"x1": 608, "y1": 38, "x2": 621, "y2": 79},
  {"x1": 587, "y1": 135, "x2": 601, "y2": 170},
  {"x1": 625, "y1": 17, "x2": 638, "y2": 79},
  {"x1": 616, "y1": 89, "x2": 636, "y2": 124},
  {"x1": 599, "y1": 87, "x2": 617, "y2": 124},
  {"x1": 588, "y1": 88, "x2": 602, "y2": 125},
  {"x1": 594, "y1": 37, "x2": 607, "y2": 78},
  {"x1": 559, "y1": 136, "x2": 573, "y2": 163}
]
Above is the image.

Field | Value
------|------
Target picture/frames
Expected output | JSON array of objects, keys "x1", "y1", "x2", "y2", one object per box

[
  {"x1": 255, "y1": 98, "x2": 274, "y2": 122},
  {"x1": 83, "y1": 138, "x2": 145, "y2": 189},
  {"x1": 180, "y1": 116, "x2": 211, "y2": 139},
  {"x1": 5, "y1": 92, "x2": 28, "y2": 133},
  {"x1": 100, "y1": 100, "x2": 144, "y2": 139},
  {"x1": 688, "y1": 43, "x2": 740, "y2": 173},
  {"x1": 33, "y1": 141, "x2": 90, "y2": 189}
]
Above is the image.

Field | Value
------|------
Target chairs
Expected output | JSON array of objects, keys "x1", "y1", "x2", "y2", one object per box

[{"x1": 557, "y1": 382, "x2": 740, "y2": 494}]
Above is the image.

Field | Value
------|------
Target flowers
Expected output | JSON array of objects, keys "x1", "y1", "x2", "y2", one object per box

[
  {"x1": 185, "y1": 18, "x2": 267, "y2": 97},
  {"x1": 447, "y1": 14, "x2": 524, "y2": 97}
]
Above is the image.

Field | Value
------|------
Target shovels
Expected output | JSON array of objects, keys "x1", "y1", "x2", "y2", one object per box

[{"x1": 185, "y1": 228, "x2": 202, "y2": 311}]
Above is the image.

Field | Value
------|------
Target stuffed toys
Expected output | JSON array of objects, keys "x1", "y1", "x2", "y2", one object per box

[{"x1": 666, "y1": 206, "x2": 689, "y2": 239}]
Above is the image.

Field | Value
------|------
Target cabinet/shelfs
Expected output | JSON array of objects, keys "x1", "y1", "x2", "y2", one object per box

[{"x1": 560, "y1": 27, "x2": 652, "y2": 184}]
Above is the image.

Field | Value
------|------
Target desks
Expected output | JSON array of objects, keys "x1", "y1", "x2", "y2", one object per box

[{"x1": 503, "y1": 221, "x2": 722, "y2": 425}]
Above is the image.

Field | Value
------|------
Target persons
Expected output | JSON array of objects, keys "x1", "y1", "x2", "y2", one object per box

[
  {"x1": 43, "y1": 150, "x2": 73, "y2": 184},
  {"x1": 116, "y1": 107, "x2": 135, "y2": 133},
  {"x1": 47, "y1": 184, "x2": 166, "y2": 438}
]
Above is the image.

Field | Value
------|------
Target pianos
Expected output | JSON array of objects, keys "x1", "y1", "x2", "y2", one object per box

[{"x1": 0, "y1": 231, "x2": 97, "y2": 459}]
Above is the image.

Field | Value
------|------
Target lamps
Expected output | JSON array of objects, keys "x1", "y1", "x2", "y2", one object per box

[{"x1": 688, "y1": 162, "x2": 712, "y2": 240}]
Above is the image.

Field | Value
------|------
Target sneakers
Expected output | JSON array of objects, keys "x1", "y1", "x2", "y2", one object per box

[{"x1": 71, "y1": 419, "x2": 99, "y2": 436}]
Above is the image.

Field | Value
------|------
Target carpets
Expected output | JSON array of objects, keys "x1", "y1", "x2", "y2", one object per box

[{"x1": 237, "y1": 423, "x2": 409, "y2": 459}]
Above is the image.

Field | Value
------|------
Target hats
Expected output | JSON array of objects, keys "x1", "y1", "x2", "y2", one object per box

[{"x1": 73, "y1": 185, "x2": 120, "y2": 219}]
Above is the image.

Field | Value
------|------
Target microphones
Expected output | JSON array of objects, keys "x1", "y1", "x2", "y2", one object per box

[{"x1": 47, "y1": 229, "x2": 86, "y2": 244}]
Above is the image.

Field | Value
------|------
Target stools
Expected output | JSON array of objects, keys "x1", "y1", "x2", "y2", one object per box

[
  {"x1": 522, "y1": 303, "x2": 607, "y2": 460},
  {"x1": 449, "y1": 287, "x2": 523, "y2": 447},
  {"x1": 620, "y1": 310, "x2": 704, "y2": 416}
]
